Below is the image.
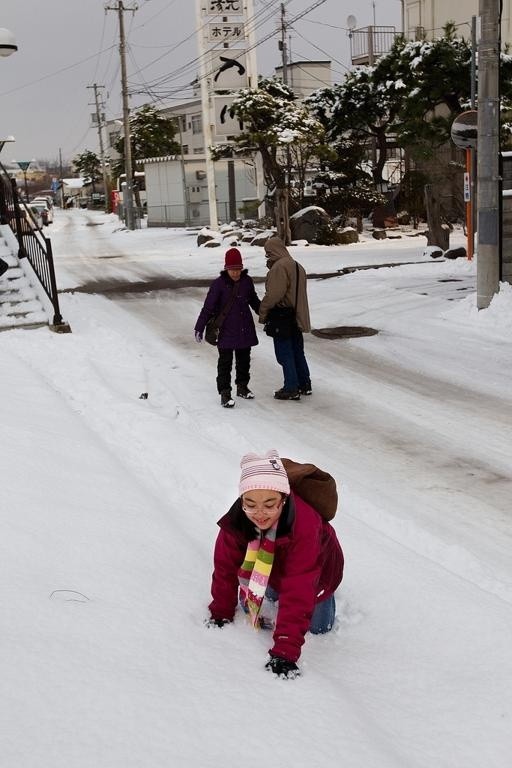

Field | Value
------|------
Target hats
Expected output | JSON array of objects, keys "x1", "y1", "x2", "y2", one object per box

[
  {"x1": 237, "y1": 448, "x2": 291, "y2": 497},
  {"x1": 225, "y1": 248, "x2": 243, "y2": 270}
]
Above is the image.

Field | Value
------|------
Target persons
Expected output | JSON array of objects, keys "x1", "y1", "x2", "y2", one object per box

[
  {"x1": 257, "y1": 236, "x2": 313, "y2": 402},
  {"x1": 193, "y1": 247, "x2": 261, "y2": 411},
  {"x1": 205, "y1": 447, "x2": 346, "y2": 684}
]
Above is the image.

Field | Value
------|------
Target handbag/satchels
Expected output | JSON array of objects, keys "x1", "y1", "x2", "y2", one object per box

[
  {"x1": 265, "y1": 306, "x2": 297, "y2": 339},
  {"x1": 205, "y1": 318, "x2": 221, "y2": 346}
]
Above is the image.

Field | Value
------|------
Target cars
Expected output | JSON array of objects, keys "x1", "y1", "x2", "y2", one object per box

[{"x1": 5, "y1": 191, "x2": 54, "y2": 236}]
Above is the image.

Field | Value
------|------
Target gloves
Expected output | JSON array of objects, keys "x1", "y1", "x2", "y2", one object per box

[
  {"x1": 265, "y1": 655, "x2": 300, "y2": 680},
  {"x1": 195, "y1": 331, "x2": 203, "y2": 343},
  {"x1": 206, "y1": 617, "x2": 233, "y2": 629}
]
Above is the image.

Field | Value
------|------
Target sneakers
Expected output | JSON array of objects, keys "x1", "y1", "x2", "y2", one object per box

[
  {"x1": 236, "y1": 384, "x2": 254, "y2": 399},
  {"x1": 274, "y1": 386, "x2": 301, "y2": 400},
  {"x1": 221, "y1": 394, "x2": 235, "y2": 407},
  {"x1": 301, "y1": 380, "x2": 312, "y2": 396}
]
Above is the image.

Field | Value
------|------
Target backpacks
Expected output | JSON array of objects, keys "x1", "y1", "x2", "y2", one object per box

[{"x1": 281, "y1": 457, "x2": 338, "y2": 521}]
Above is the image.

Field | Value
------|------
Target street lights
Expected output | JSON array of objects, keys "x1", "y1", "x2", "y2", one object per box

[{"x1": 15, "y1": 160, "x2": 32, "y2": 202}]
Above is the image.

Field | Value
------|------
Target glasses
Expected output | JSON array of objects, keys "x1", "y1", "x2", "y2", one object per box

[{"x1": 242, "y1": 499, "x2": 283, "y2": 514}]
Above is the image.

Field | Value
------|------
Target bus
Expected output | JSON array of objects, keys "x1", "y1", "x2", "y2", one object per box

[{"x1": 112, "y1": 170, "x2": 150, "y2": 216}]
